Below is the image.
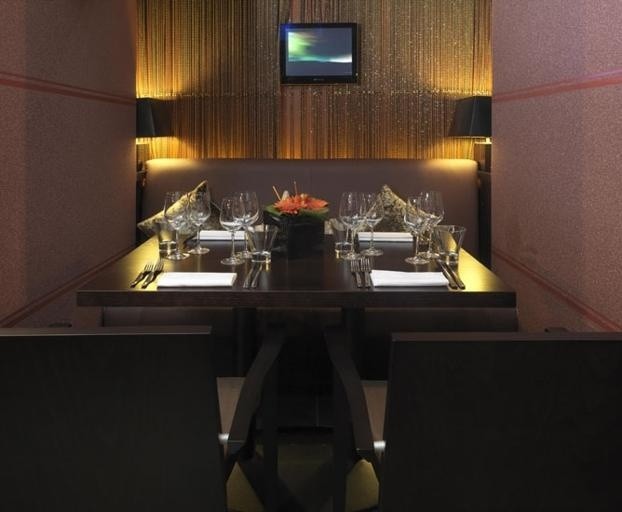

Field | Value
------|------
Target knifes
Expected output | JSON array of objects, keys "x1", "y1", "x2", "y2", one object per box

[
  {"x1": 250, "y1": 257, "x2": 267, "y2": 289},
  {"x1": 242, "y1": 260, "x2": 256, "y2": 289},
  {"x1": 445, "y1": 261, "x2": 465, "y2": 289},
  {"x1": 436, "y1": 259, "x2": 458, "y2": 290}
]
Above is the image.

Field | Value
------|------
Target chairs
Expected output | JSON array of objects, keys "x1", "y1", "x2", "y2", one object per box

[
  {"x1": 0, "y1": 323, "x2": 231, "y2": 512},
  {"x1": 386, "y1": 329, "x2": 619, "y2": 512}
]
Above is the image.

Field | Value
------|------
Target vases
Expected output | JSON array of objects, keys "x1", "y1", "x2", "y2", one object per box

[{"x1": 282, "y1": 224, "x2": 313, "y2": 251}]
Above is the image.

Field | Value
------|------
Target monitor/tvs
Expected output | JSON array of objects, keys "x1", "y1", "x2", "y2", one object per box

[{"x1": 279, "y1": 22, "x2": 360, "y2": 87}]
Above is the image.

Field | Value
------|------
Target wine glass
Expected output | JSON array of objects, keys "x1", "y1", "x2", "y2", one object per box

[
  {"x1": 220, "y1": 196, "x2": 245, "y2": 267},
  {"x1": 233, "y1": 191, "x2": 260, "y2": 261},
  {"x1": 342, "y1": 194, "x2": 366, "y2": 261},
  {"x1": 184, "y1": 193, "x2": 212, "y2": 255},
  {"x1": 336, "y1": 191, "x2": 358, "y2": 251},
  {"x1": 360, "y1": 193, "x2": 384, "y2": 256},
  {"x1": 162, "y1": 191, "x2": 192, "y2": 261},
  {"x1": 403, "y1": 196, "x2": 431, "y2": 265},
  {"x1": 418, "y1": 191, "x2": 444, "y2": 258}
]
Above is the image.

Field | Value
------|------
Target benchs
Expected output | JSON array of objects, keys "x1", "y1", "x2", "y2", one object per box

[{"x1": 129, "y1": 155, "x2": 519, "y2": 376}]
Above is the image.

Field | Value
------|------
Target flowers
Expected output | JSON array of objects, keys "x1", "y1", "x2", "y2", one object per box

[{"x1": 263, "y1": 180, "x2": 331, "y2": 225}]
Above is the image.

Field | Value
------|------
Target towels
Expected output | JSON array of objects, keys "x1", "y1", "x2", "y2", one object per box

[
  {"x1": 370, "y1": 270, "x2": 450, "y2": 287},
  {"x1": 193, "y1": 230, "x2": 248, "y2": 240},
  {"x1": 158, "y1": 272, "x2": 237, "y2": 288},
  {"x1": 358, "y1": 232, "x2": 413, "y2": 243}
]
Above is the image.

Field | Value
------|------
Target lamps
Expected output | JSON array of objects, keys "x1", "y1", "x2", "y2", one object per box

[
  {"x1": 137, "y1": 97, "x2": 176, "y2": 169},
  {"x1": 450, "y1": 96, "x2": 490, "y2": 173}
]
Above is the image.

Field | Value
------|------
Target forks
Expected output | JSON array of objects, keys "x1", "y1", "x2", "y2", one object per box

[
  {"x1": 141, "y1": 261, "x2": 165, "y2": 288},
  {"x1": 360, "y1": 257, "x2": 372, "y2": 289},
  {"x1": 349, "y1": 259, "x2": 363, "y2": 288},
  {"x1": 130, "y1": 264, "x2": 153, "y2": 287}
]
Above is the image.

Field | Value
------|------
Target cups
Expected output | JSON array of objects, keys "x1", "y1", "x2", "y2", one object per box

[
  {"x1": 247, "y1": 225, "x2": 279, "y2": 261},
  {"x1": 330, "y1": 219, "x2": 355, "y2": 253},
  {"x1": 431, "y1": 226, "x2": 467, "y2": 265},
  {"x1": 155, "y1": 219, "x2": 178, "y2": 254}
]
